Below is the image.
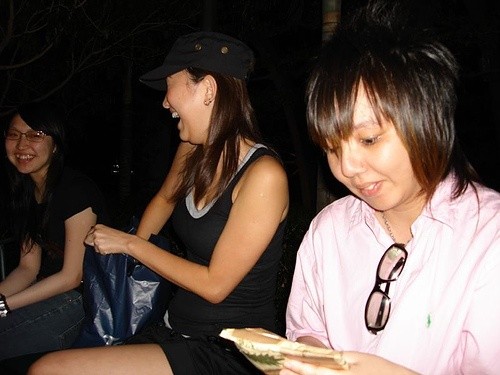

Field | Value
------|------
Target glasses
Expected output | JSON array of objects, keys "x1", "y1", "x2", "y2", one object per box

[
  {"x1": 6, "y1": 129, "x2": 52, "y2": 143},
  {"x1": 364, "y1": 242, "x2": 407, "y2": 336}
]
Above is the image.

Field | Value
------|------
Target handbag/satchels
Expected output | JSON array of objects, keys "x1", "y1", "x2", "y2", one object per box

[{"x1": 71, "y1": 235, "x2": 175, "y2": 349}]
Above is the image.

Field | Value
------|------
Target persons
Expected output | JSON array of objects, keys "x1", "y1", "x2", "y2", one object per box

[
  {"x1": 0, "y1": 95, "x2": 108, "y2": 364},
  {"x1": 26, "y1": 31, "x2": 295, "y2": 375},
  {"x1": 283, "y1": 0, "x2": 500, "y2": 375}
]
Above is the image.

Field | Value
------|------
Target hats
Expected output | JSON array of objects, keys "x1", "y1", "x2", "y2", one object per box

[{"x1": 139, "y1": 32, "x2": 253, "y2": 91}]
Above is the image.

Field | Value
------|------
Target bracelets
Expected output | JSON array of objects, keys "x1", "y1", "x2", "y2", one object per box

[{"x1": 0, "y1": 294, "x2": 10, "y2": 319}]
